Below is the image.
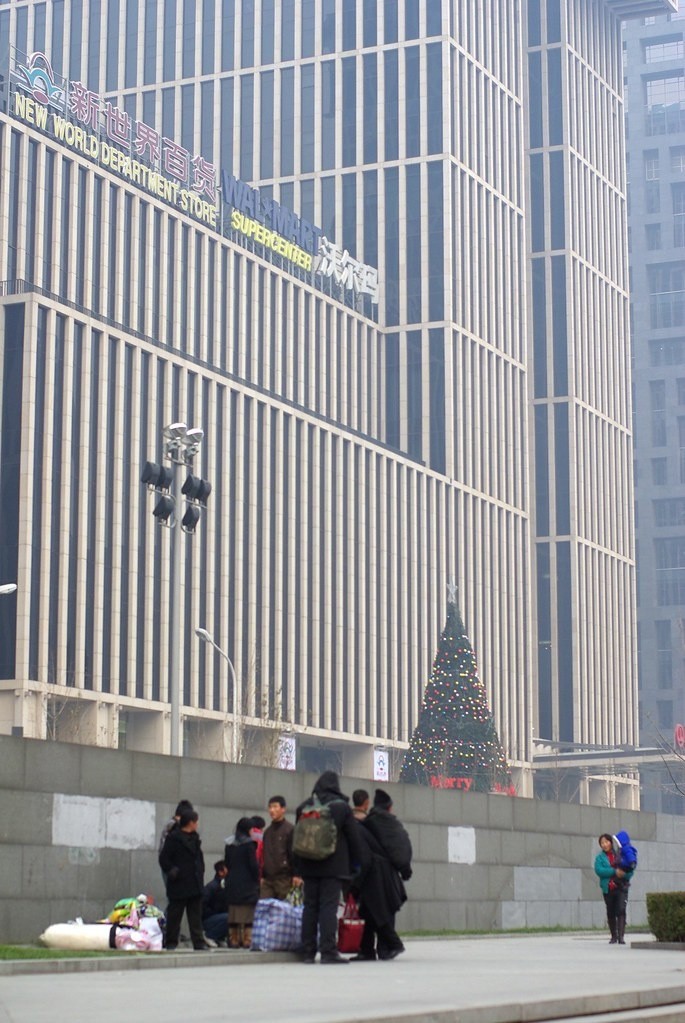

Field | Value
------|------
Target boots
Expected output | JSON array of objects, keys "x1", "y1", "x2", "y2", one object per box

[
  {"x1": 618, "y1": 916, "x2": 626, "y2": 943},
  {"x1": 243, "y1": 927, "x2": 252, "y2": 949},
  {"x1": 229, "y1": 928, "x2": 239, "y2": 947},
  {"x1": 608, "y1": 918, "x2": 618, "y2": 943}
]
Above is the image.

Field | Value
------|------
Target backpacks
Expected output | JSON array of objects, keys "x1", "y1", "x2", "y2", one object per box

[{"x1": 292, "y1": 792, "x2": 346, "y2": 860}]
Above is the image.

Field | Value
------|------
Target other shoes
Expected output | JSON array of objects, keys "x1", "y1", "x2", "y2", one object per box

[
  {"x1": 202, "y1": 930, "x2": 217, "y2": 947},
  {"x1": 320, "y1": 955, "x2": 348, "y2": 963},
  {"x1": 349, "y1": 952, "x2": 376, "y2": 961},
  {"x1": 304, "y1": 956, "x2": 315, "y2": 963},
  {"x1": 194, "y1": 942, "x2": 207, "y2": 949},
  {"x1": 383, "y1": 947, "x2": 404, "y2": 959},
  {"x1": 217, "y1": 939, "x2": 228, "y2": 947},
  {"x1": 167, "y1": 944, "x2": 176, "y2": 949}
]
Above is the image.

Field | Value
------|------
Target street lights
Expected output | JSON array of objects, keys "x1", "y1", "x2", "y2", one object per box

[
  {"x1": 140, "y1": 423, "x2": 212, "y2": 757},
  {"x1": 194, "y1": 627, "x2": 238, "y2": 764}
]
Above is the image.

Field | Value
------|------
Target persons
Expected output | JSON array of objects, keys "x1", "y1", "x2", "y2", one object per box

[
  {"x1": 158, "y1": 770, "x2": 413, "y2": 964},
  {"x1": 594, "y1": 830, "x2": 638, "y2": 944}
]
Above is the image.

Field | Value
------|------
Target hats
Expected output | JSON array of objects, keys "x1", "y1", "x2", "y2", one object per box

[{"x1": 374, "y1": 789, "x2": 392, "y2": 810}]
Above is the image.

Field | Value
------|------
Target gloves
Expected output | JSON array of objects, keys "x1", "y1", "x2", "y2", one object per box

[{"x1": 402, "y1": 869, "x2": 412, "y2": 881}]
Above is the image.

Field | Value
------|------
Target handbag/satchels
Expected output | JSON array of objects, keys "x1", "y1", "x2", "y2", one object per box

[
  {"x1": 337, "y1": 893, "x2": 365, "y2": 952},
  {"x1": 250, "y1": 888, "x2": 321, "y2": 952}
]
